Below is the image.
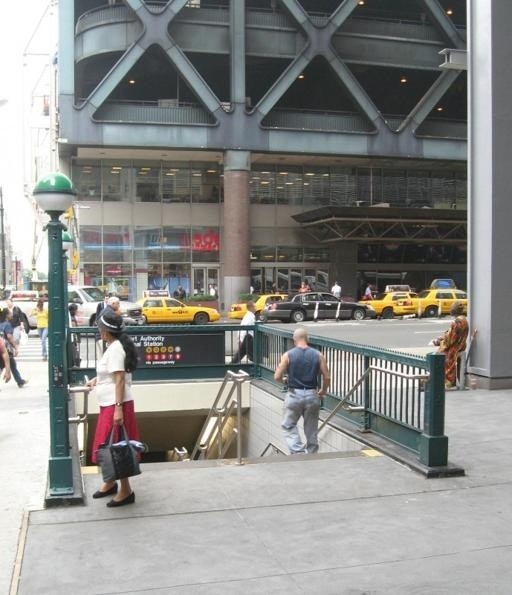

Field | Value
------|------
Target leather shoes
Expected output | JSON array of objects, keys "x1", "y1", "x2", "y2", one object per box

[
  {"x1": 106, "y1": 491, "x2": 135, "y2": 507},
  {"x1": 92, "y1": 483, "x2": 118, "y2": 498}
]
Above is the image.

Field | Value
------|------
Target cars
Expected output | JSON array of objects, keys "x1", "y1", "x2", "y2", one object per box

[
  {"x1": 227, "y1": 291, "x2": 289, "y2": 322},
  {"x1": 395, "y1": 277, "x2": 467, "y2": 319},
  {"x1": 132, "y1": 295, "x2": 222, "y2": 325},
  {"x1": 264, "y1": 291, "x2": 377, "y2": 322},
  {"x1": 354, "y1": 284, "x2": 421, "y2": 320}
]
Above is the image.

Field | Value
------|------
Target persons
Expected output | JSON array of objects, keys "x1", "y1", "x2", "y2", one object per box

[
  {"x1": 331, "y1": 281, "x2": 341, "y2": 298},
  {"x1": 0, "y1": 295, "x2": 122, "y2": 388},
  {"x1": 85, "y1": 311, "x2": 142, "y2": 508},
  {"x1": 299, "y1": 282, "x2": 308, "y2": 292},
  {"x1": 273, "y1": 328, "x2": 330, "y2": 454},
  {"x1": 172, "y1": 285, "x2": 186, "y2": 301},
  {"x1": 418, "y1": 302, "x2": 468, "y2": 391},
  {"x1": 365, "y1": 284, "x2": 374, "y2": 299},
  {"x1": 229, "y1": 300, "x2": 262, "y2": 363},
  {"x1": 208, "y1": 283, "x2": 215, "y2": 296}
]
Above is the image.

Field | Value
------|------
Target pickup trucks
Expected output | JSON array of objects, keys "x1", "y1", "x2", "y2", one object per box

[{"x1": 9, "y1": 283, "x2": 144, "y2": 334}]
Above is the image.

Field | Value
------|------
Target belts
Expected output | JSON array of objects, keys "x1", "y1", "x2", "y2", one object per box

[{"x1": 291, "y1": 385, "x2": 316, "y2": 390}]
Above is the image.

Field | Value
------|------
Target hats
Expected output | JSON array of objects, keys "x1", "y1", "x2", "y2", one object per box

[{"x1": 96, "y1": 312, "x2": 127, "y2": 333}]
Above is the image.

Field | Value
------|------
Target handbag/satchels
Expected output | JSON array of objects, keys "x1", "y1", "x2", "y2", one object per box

[
  {"x1": 95, "y1": 423, "x2": 142, "y2": 483},
  {"x1": 3, "y1": 337, "x2": 14, "y2": 358}
]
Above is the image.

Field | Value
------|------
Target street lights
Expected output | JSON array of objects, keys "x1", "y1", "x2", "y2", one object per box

[
  {"x1": 61, "y1": 230, "x2": 75, "y2": 328},
  {"x1": 29, "y1": 166, "x2": 79, "y2": 494}
]
